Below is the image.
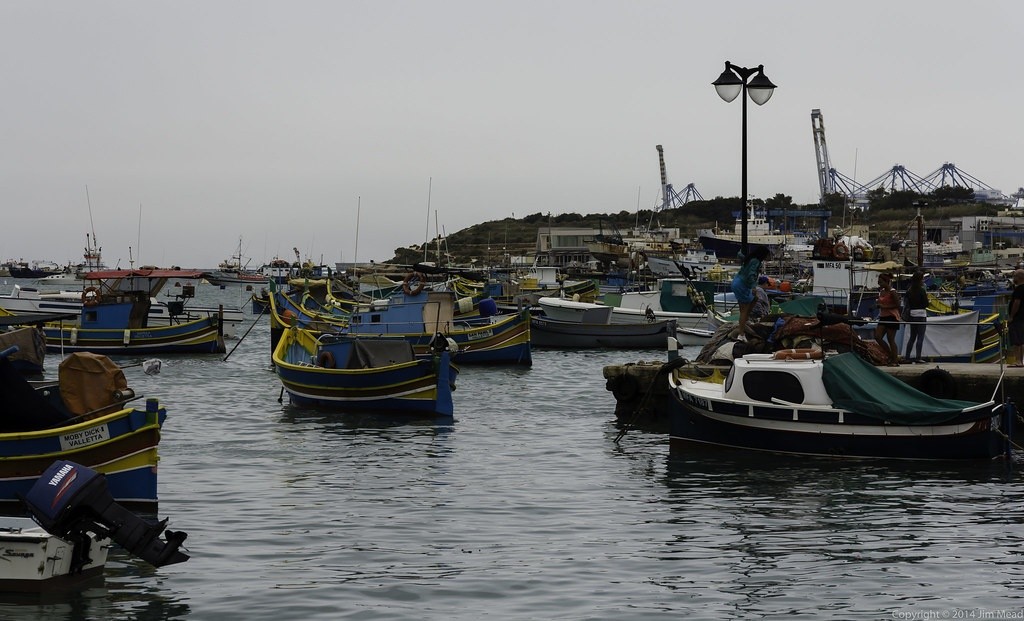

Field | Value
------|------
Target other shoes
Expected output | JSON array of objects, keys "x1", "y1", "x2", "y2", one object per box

[
  {"x1": 887, "y1": 360, "x2": 898, "y2": 367},
  {"x1": 736, "y1": 334, "x2": 747, "y2": 343},
  {"x1": 915, "y1": 359, "x2": 926, "y2": 364},
  {"x1": 899, "y1": 359, "x2": 911, "y2": 364},
  {"x1": 887, "y1": 351, "x2": 892, "y2": 363}
]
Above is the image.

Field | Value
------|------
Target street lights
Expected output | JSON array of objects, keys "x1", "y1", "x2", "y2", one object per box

[{"x1": 712, "y1": 62, "x2": 778, "y2": 264}]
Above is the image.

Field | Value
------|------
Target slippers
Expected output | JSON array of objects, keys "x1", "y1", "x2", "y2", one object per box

[{"x1": 1007, "y1": 363, "x2": 1024, "y2": 367}]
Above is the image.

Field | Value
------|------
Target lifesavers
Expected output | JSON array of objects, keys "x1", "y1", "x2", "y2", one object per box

[
  {"x1": 833, "y1": 243, "x2": 849, "y2": 260},
  {"x1": 317, "y1": 350, "x2": 336, "y2": 368},
  {"x1": 774, "y1": 348, "x2": 825, "y2": 360},
  {"x1": 853, "y1": 244, "x2": 866, "y2": 261},
  {"x1": 704, "y1": 255, "x2": 709, "y2": 260},
  {"x1": 82, "y1": 287, "x2": 101, "y2": 306},
  {"x1": 402, "y1": 272, "x2": 426, "y2": 296}
]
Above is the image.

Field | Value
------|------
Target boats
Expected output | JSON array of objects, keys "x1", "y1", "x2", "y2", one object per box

[
  {"x1": 0, "y1": 238, "x2": 243, "y2": 356},
  {"x1": 243, "y1": 217, "x2": 1024, "y2": 478},
  {"x1": 1, "y1": 349, "x2": 168, "y2": 517},
  {"x1": 0, "y1": 461, "x2": 190, "y2": 598}
]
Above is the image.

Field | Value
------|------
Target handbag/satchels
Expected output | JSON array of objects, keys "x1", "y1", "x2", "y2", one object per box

[{"x1": 901, "y1": 292, "x2": 909, "y2": 321}]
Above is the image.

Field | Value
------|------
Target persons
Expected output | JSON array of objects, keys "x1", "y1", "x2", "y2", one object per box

[
  {"x1": 731, "y1": 247, "x2": 770, "y2": 342},
  {"x1": 751, "y1": 276, "x2": 769, "y2": 318},
  {"x1": 904, "y1": 272, "x2": 929, "y2": 364},
  {"x1": 1006, "y1": 269, "x2": 1024, "y2": 367},
  {"x1": 868, "y1": 273, "x2": 902, "y2": 367}
]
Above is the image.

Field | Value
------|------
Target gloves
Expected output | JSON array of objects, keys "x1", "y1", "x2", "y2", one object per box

[{"x1": 869, "y1": 304, "x2": 877, "y2": 312}]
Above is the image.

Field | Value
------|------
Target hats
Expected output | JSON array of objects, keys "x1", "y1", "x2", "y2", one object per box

[{"x1": 758, "y1": 276, "x2": 772, "y2": 288}]
